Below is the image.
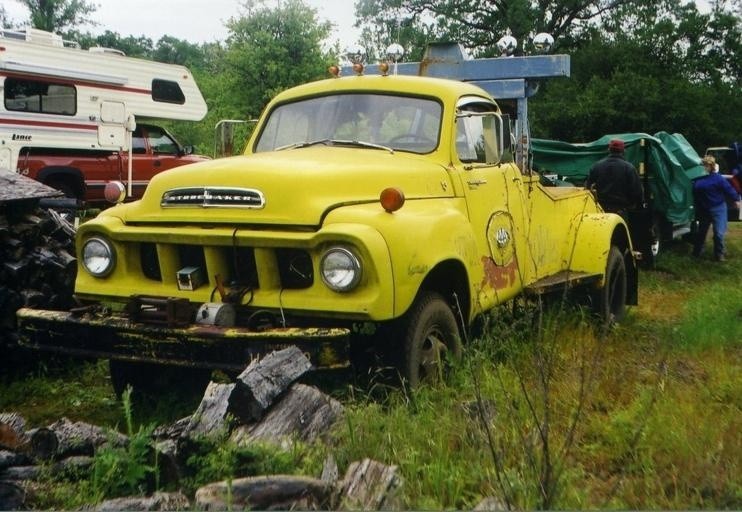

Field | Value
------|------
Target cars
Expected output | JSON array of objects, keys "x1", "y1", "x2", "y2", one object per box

[{"x1": 528, "y1": 131, "x2": 700, "y2": 264}]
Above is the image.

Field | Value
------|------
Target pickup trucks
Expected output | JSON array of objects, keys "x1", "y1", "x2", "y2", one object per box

[{"x1": 16, "y1": 44, "x2": 639, "y2": 394}]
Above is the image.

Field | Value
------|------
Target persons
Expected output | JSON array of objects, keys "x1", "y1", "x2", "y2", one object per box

[
  {"x1": 580, "y1": 139, "x2": 645, "y2": 234},
  {"x1": 690, "y1": 155, "x2": 742, "y2": 263}
]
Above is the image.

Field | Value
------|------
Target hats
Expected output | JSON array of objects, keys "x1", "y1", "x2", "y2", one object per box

[{"x1": 608, "y1": 140, "x2": 625, "y2": 151}]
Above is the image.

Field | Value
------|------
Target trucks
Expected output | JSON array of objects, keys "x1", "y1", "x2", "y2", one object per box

[{"x1": 1, "y1": 23, "x2": 226, "y2": 204}]
